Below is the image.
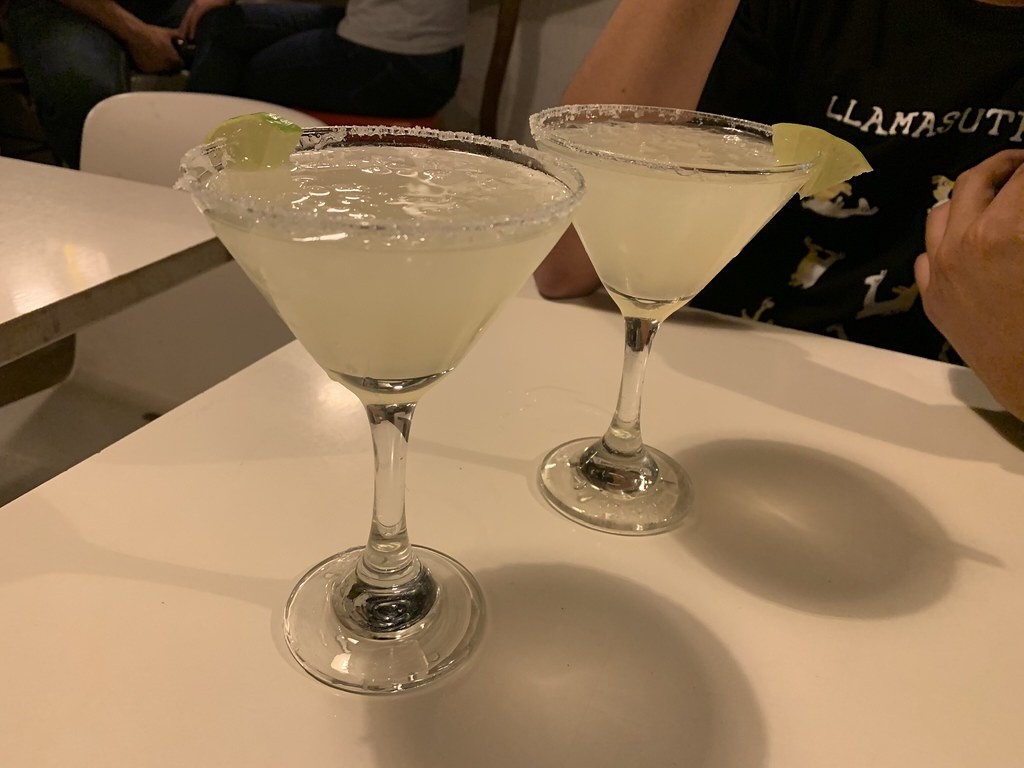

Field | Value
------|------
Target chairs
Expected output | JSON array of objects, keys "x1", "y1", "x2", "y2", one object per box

[{"x1": 0, "y1": 90, "x2": 326, "y2": 512}]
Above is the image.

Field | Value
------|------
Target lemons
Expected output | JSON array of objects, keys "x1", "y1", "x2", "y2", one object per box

[
  {"x1": 207, "y1": 111, "x2": 304, "y2": 167},
  {"x1": 773, "y1": 122, "x2": 874, "y2": 200}
]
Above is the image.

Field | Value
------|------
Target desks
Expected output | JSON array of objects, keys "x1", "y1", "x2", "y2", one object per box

[
  {"x1": 0, "y1": 154, "x2": 234, "y2": 369},
  {"x1": 0, "y1": 278, "x2": 1024, "y2": 768}
]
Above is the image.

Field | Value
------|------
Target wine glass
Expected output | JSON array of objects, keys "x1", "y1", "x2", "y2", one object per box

[
  {"x1": 529, "y1": 102, "x2": 824, "y2": 537},
  {"x1": 182, "y1": 126, "x2": 589, "y2": 699}
]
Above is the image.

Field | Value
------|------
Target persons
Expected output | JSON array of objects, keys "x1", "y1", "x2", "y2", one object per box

[
  {"x1": 0, "y1": 0, "x2": 264, "y2": 171},
  {"x1": 193, "y1": 0, "x2": 470, "y2": 122},
  {"x1": 530, "y1": 1, "x2": 1024, "y2": 434}
]
